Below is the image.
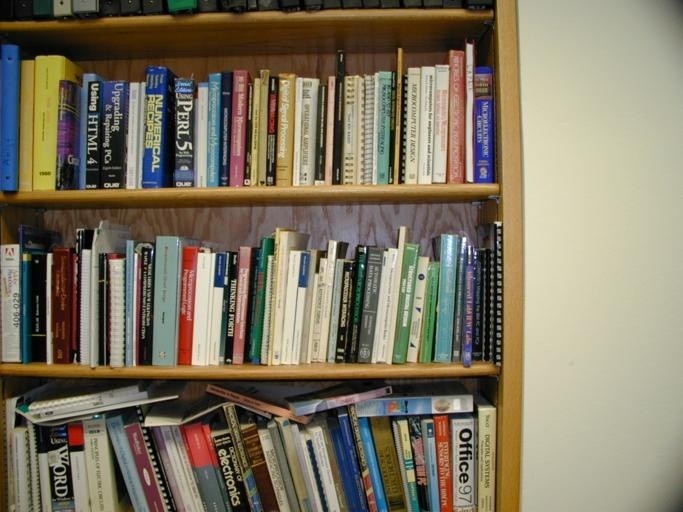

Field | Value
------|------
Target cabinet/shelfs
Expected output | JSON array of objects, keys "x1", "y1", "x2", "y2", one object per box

[{"x1": 0, "y1": 0, "x2": 525, "y2": 512}]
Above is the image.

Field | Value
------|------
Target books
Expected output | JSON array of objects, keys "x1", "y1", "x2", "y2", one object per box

[
  {"x1": 0, "y1": 377, "x2": 496, "y2": 512},
  {"x1": 1, "y1": 45, "x2": 495, "y2": 192},
  {"x1": 0, "y1": 0, "x2": 495, "y2": 20},
  {"x1": 0, "y1": 219, "x2": 503, "y2": 373}
]
